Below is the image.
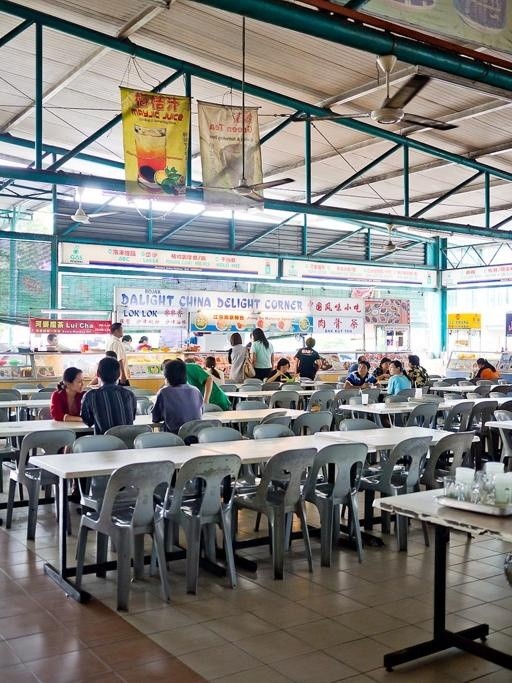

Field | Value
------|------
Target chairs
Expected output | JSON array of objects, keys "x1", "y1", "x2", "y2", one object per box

[{"x1": 0, "y1": 375, "x2": 512, "y2": 613}]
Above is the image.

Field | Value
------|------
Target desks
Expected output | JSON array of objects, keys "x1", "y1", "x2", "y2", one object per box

[{"x1": 372, "y1": 488, "x2": 512, "y2": 671}]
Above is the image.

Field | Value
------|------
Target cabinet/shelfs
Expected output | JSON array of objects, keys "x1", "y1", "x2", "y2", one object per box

[
  {"x1": 126, "y1": 352, "x2": 231, "y2": 379},
  {"x1": 446, "y1": 351, "x2": 502, "y2": 371}
]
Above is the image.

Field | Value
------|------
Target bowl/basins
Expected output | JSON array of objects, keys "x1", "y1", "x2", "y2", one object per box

[
  {"x1": 286, "y1": 378, "x2": 294, "y2": 382},
  {"x1": 320, "y1": 364, "x2": 332, "y2": 369}
]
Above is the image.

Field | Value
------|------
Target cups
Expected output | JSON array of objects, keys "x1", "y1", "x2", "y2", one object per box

[
  {"x1": 440, "y1": 458, "x2": 512, "y2": 506},
  {"x1": 80, "y1": 343, "x2": 90, "y2": 352},
  {"x1": 362, "y1": 393, "x2": 369, "y2": 404},
  {"x1": 415, "y1": 387, "x2": 423, "y2": 399}
]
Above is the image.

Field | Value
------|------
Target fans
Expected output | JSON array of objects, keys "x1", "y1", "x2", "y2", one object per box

[
  {"x1": 196, "y1": 15, "x2": 295, "y2": 202},
  {"x1": 371, "y1": 224, "x2": 408, "y2": 252},
  {"x1": 293, "y1": 54, "x2": 458, "y2": 130},
  {"x1": 28, "y1": 187, "x2": 126, "y2": 224}
]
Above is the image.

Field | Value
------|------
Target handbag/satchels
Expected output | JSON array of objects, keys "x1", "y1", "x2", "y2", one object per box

[{"x1": 244, "y1": 360, "x2": 256, "y2": 378}]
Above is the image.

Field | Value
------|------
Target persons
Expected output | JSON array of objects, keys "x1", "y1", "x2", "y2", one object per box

[
  {"x1": 122, "y1": 335, "x2": 135, "y2": 352},
  {"x1": 343, "y1": 354, "x2": 430, "y2": 396},
  {"x1": 105, "y1": 323, "x2": 131, "y2": 386},
  {"x1": 50, "y1": 368, "x2": 88, "y2": 498},
  {"x1": 87, "y1": 351, "x2": 120, "y2": 388},
  {"x1": 137, "y1": 336, "x2": 151, "y2": 350},
  {"x1": 151, "y1": 328, "x2": 322, "y2": 434},
  {"x1": 77, "y1": 357, "x2": 137, "y2": 516},
  {"x1": 474, "y1": 358, "x2": 500, "y2": 381},
  {"x1": 47, "y1": 334, "x2": 58, "y2": 346}
]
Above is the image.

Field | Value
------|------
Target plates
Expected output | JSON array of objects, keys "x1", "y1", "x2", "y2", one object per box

[
  {"x1": 276, "y1": 320, "x2": 290, "y2": 331},
  {"x1": 332, "y1": 360, "x2": 343, "y2": 370},
  {"x1": 235, "y1": 320, "x2": 246, "y2": 331},
  {"x1": 255, "y1": 319, "x2": 270, "y2": 331},
  {"x1": 305, "y1": 380, "x2": 314, "y2": 384},
  {"x1": 299, "y1": 317, "x2": 311, "y2": 330},
  {"x1": 194, "y1": 322, "x2": 207, "y2": 330},
  {"x1": 46, "y1": 345, "x2": 56, "y2": 351},
  {"x1": 366, "y1": 298, "x2": 401, "y2": 323},
  {"x1": 215, "y1": 318, "x2": 229, "y2": 331}
]
